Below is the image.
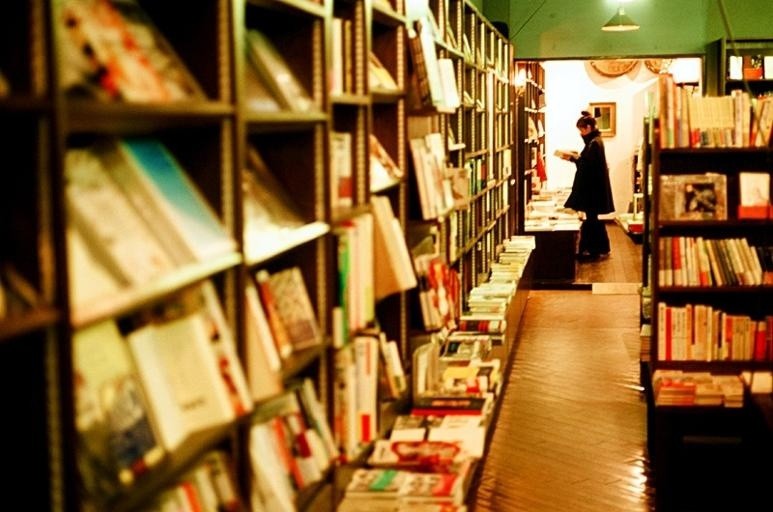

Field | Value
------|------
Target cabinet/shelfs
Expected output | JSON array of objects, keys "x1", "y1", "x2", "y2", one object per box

[
  {"x1": 517, "y1": 61, "x2": 594, "y2": 291},
  {"x1": 632, "y1": 38, "x2": 773, "y2": 512},
  {"x1": 1, "y1": 0, "x2": 536, "y2": 510}
]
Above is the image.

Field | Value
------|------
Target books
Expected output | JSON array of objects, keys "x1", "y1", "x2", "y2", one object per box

[
  {"x1": 528, "y1": 67, "x2": 547, "y2": 193},
  {"x1": 332, "y1": 1, "x2": 512, "y2": 464},
  {"x1": 52, "y1": 0, "x2": 211, "y2": 103},
  {"x1": 64, "y1": 137, "x2": 239, "y2": 318},
  {"x1": 658, "y1": 235, "x2": 773, "y2": 287},
  {"x1": 658, "y1": 301, "x2": 773, "y2": 362},
  {"x1": 337, "y1": 235, "x2": 536, "y2": 512},
  {"x1": 245, "y1": 265, "x2": 323, "y2": 404},
  {"x1": 659, "y1": 72, "x2": 773, "y2": 149},
  {"x1": 652, "y1": 369, "x2": 773, "y2": 407},
  {"x1": 243, "y1": 144, "x2": 309, "y2": 245},
  {"x1": 554, "y1": 149, "x2": 578, "y2": 160},
  {"x1": 247, "y1": 376, "x2": 340, "y2": 512},
  {"x1": 243, "y1": 28, "x2": 319, "y2": 114},
  {"x1": 658, "y1": 172, "x2": 773, "y2": 220},
  {"x1": 616, "y1": 73, "x2": 659, "y2": 361},
  {"x1": 524, "y1": 185, "x2": 585, "y2": 231},
  {"x1": 71, "y1": 278, "x2": 254, "y2": 512}
]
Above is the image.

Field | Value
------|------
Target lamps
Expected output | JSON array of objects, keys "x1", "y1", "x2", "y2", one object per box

[{"x1": 601, "y1": 0, "x2": 640, "y2": 30}]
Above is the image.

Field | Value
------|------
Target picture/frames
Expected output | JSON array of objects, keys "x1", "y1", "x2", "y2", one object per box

[{"x1": 589, "y1": 102, "x2": 616, "y2": 136}]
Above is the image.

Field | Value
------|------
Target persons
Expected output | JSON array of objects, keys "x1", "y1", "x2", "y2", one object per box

[{"x1": 560, "y1": 110, "x2": 616, "y2": 260}]
